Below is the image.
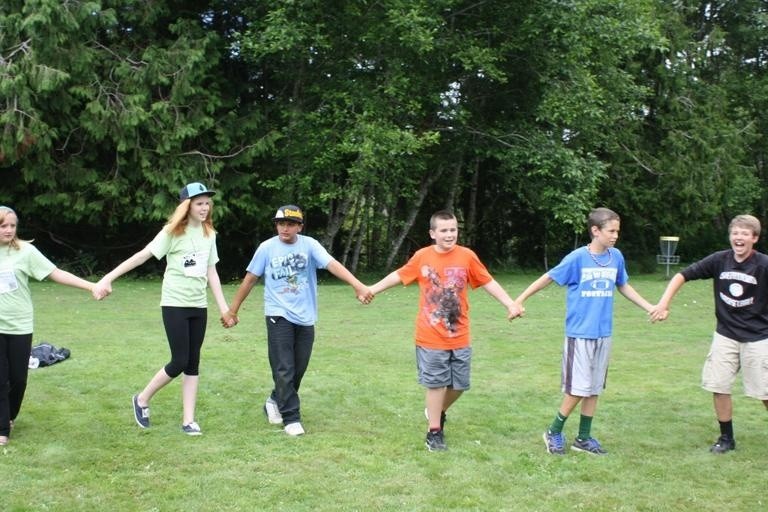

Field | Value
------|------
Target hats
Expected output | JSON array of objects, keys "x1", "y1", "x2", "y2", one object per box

[
  {"x1": 179, "y1": 182, "x2": 216, "y2": 202},
  {"x1": 272, "y1": 205, "x2": 303, "y2": 223}
]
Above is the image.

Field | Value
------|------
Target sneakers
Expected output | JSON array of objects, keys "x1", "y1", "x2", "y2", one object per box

[
  {"x1": 426, "y1": 430, "x2": 446, "y2": 453},
  {"x1": 182, "y1": 422, "x2": 202, "y2": 436},
  {"x1": 542, "y1": 430, "x2": 608, "y2": 455},
  {"x1": 132, "y1": 395, "x2": 149, "y2": 429},
  {"x1": 710, "y1": 434, "x2": 735, "y2": 453},
  {"x1": 264, "y1": 397, "x2": 305, "y2": 436}
]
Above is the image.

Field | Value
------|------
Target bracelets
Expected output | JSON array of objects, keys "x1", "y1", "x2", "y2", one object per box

[{"x1": 227, "y1": 310, "x2": 237, "y2": 317}]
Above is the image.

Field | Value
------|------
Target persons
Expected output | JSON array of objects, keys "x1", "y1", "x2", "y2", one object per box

[
  {"x1": 360, "y1": 210, "x2": 523, "y2": 454},
  {"x1": 92, "y1": 183, "x2": 239, "y2": 437},
  {"x1": 509, "y1": 206, "x2": 669, "y2": 457},
  {"x1": 647, "y1": 214, "x2": 767, "y2": 455},
  {"x1": 221, "y1": 204, "x2": 374, "y2": 436},
  {"x1": 0, "y1": 206, "x2": 112, "y2": 445}
]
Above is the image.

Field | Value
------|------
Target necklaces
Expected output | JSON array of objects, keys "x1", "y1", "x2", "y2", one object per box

[{"x1": 588, "y1": 243, "x2": 613, "y2": 267}]
